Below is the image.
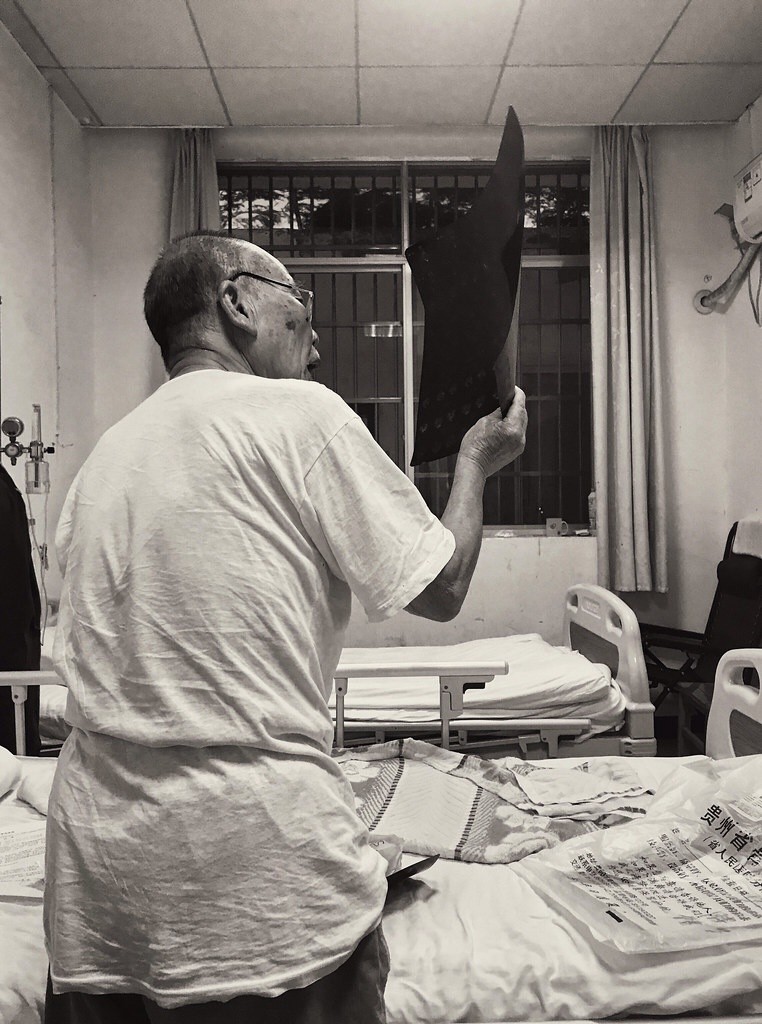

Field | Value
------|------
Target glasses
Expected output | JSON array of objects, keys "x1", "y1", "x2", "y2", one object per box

[{"x1": 226, "y1": 272, "x2": 314, "y2": 322}]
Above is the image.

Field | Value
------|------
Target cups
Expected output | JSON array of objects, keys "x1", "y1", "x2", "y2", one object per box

[{"x1": 546, "y1": 518, "x2": 569, "y2": 538}]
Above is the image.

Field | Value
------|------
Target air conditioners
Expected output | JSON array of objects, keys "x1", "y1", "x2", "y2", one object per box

[{"x1": 733, "y1": 153, "x2": 762, "y2": 244}]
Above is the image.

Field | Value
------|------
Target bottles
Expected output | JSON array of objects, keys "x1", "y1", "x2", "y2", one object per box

[{"x1": 588, "y1": 488, "x2": 597, "y2": 538}]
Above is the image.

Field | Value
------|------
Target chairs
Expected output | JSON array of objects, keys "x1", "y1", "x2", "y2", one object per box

[{"x1": 638, "y1": 521, "x2": 762, "y2": 716}]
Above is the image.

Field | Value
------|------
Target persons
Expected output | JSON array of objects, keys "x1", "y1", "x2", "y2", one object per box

[
  {"x1": 43, "y1": 230, "x2": 529, "y2": 1023},
  {"x1": 0, "y1": 464, "x2": 42, "y2": 757}
]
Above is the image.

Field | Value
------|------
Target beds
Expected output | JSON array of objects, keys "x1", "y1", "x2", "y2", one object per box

[{"x1": 0, "y1": 582, "x2": 762, "y2": 1024}]
